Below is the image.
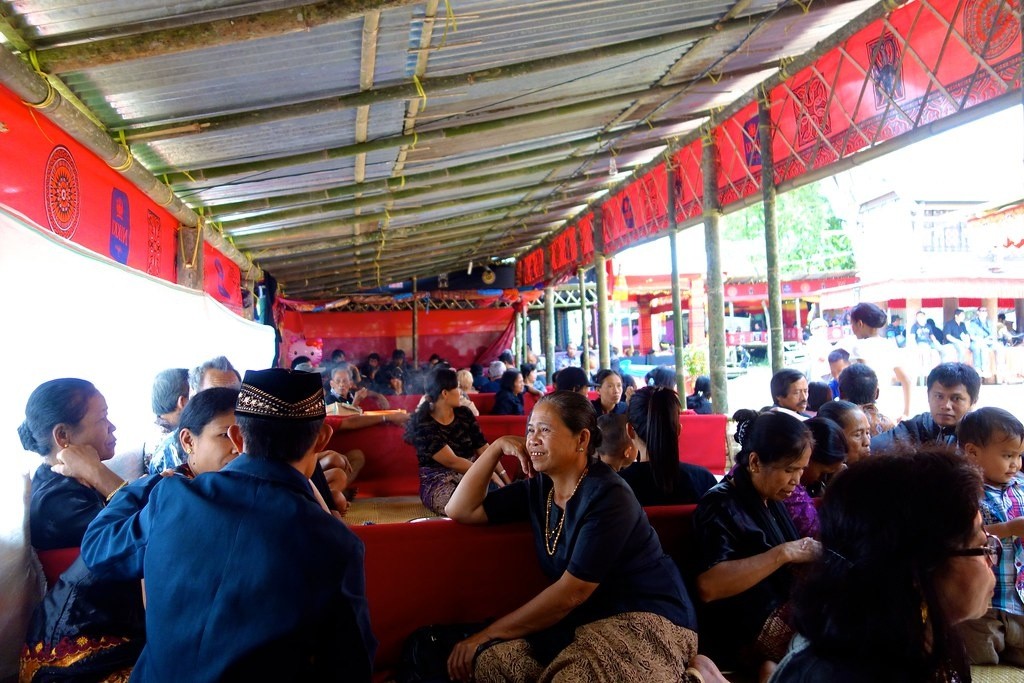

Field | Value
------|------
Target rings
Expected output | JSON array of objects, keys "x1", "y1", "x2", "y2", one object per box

[
  {"x1": 804, "y1": 541, "x2": 807, "y2": 543},
  {"x1": 802, "y1": 546, "x2": 804, "y2": 549}
]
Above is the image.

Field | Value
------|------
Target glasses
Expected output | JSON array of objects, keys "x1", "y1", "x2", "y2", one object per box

[{"x1": 938, "y1": 531, "x2": 1004, "y2": 567}]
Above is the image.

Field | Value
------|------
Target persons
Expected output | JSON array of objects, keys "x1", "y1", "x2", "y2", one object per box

[
  {"x1": 16, "y1": 347, "x2": 1024, "y2": 683},
  {"x1": 526, "y1": 302, "x2": 1024, "y2": 421}
]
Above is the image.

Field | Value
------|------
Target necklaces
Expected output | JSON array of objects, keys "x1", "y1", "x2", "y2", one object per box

[
  {"x1": 545, "y1": 466, "x2": 587, "y2": 555},
  {"x1": 547, "y1": 472, "x2": 586, "y2": 540}
]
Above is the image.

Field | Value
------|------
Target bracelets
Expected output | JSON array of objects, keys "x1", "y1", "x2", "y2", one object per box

[
  {"x1": 106, "y1": 481, "x2": 128, "y2": 502},
  {"x1": 499, "y1": 470, "x2": 506, "y2": 475},
  {"x1": 383, "y1": 415, "x2": 385, "y2": 421}
]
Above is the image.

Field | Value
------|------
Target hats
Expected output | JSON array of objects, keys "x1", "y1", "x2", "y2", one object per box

[
  {"x1": 556, "y1": 367, "x2": 601, "y2": 390},
  {"x1": 234, "y1": 369, "x2": 328, "y2": 419}
]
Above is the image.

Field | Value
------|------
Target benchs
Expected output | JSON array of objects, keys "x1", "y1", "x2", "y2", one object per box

[{"x1": 29, "y1": 395, "x2": 819, "y2": 683}]
